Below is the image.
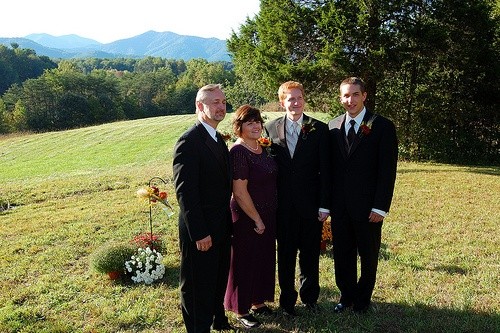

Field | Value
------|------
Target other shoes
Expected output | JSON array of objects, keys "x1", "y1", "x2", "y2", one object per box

[
  {"x1": 239, "y1": 314, "x2": 258, "y2": 328},
  {"x1": 351, "y1": 299, "x2": 370, "y2": 314},
  {"x1": 333, "y1": 300, "x2": 350, "y2": 313},
  {"x1": 213, "y1": 322, "x2": 235, "y2": 333},
  {"x1": 307, "y1": 301, "x2": 321, "y2": 312},
  {"x1": 253, "y1": 306, "x2": 273, "y2": 316},
  {"x1": 282, "y1": 304, "x2": 294, "y2": 316}
]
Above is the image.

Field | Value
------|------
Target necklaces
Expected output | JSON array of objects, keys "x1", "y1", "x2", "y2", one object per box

[{"x1": 242, "y1": 139, "x2": 260, "y2": 151}]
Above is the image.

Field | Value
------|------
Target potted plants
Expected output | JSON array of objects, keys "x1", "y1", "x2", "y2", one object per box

[{"x1": 92, "y1": 246, "x2": 134, "y2": 280}]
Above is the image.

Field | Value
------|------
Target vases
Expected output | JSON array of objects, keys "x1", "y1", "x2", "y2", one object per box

[{"x1": 321, "y1": 241, "x2": 327, "y2": 255}]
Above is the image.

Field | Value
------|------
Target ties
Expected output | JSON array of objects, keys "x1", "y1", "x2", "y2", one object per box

[
  {"x1": 288, "y1": 122, "x2": 297, "y2": 159},
  {"x1": 214, "y1": 131, "x2": 224, "y2": 145},
  {"x1": 347, "y1": 119, "x2": 356, "y2": 146}
]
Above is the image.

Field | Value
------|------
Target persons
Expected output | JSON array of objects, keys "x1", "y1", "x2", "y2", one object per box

[
  {"x1": 262, "y1": 81, "x2": 330, "y2": 319},
  {"x1": 172, "y1": 84, "x2": 233, "y2": 333},
  {"x1": 327, "y1": 77, "x2": 398, "y2": 314},
  {"x1": 224, "y1": 105, "x2": 277, "y2": 329}
]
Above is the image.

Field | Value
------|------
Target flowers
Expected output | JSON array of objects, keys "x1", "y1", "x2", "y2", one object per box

[
  {"x1": 322, "y1": 216, "x2": 333, "y2": 242},
  {"x1": 126, "y1": 247, "x2": 165, "y2": 285},
  {"x1": 131, "y1": 234, "x2": 167, "y2": 253},
  {"x1": 302, "y1": 118, "x2": 316, "y2": 140},
  {"x1": 221, "y1": 130, "x2": 232, "y2": 143},
  {"x1": 137, "y1": 187, "x2": 173, "y2": 210},
  {"x1": 259, "y1": 137, "x2": 272, "y2": 147},
  {"x1": 359, "y1": 116, "x2": 376, "y2": 140}
]
